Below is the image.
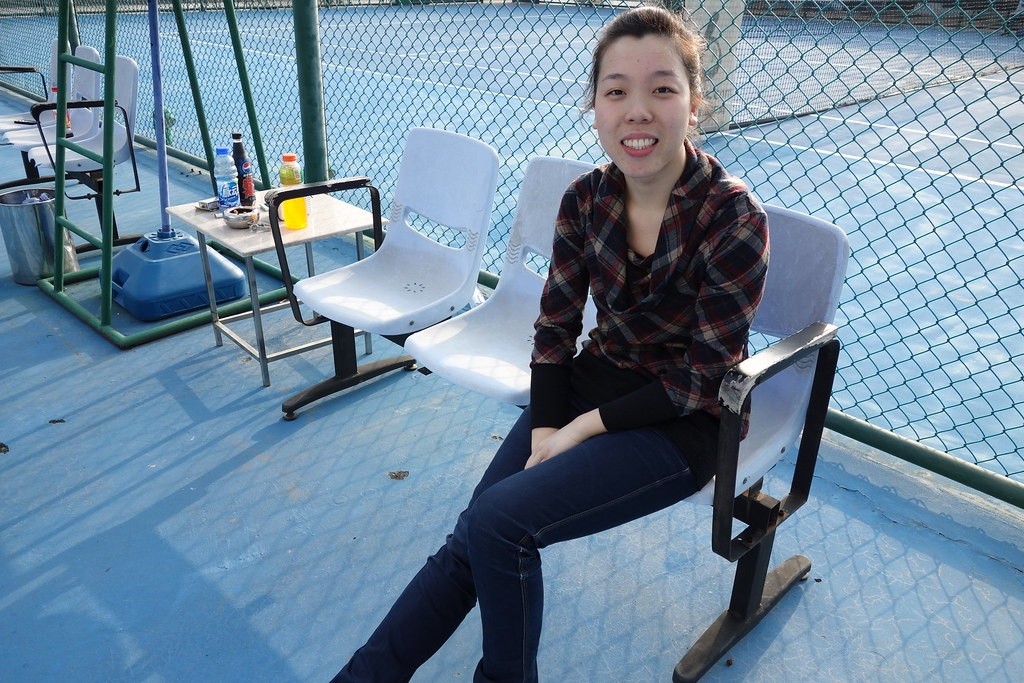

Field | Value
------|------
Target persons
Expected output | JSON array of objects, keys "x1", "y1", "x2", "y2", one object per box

[{"x1": 329, "y1": 6, "x2": 770, "y2": 683}]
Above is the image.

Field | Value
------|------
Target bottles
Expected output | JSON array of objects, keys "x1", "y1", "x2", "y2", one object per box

[
  {"x1": 280, "y1": 153, "x2": 308, "y2": 230},
  {"x1": 214, "y1": 148, "x2": 242, "y2": 210},
  {"x1": 232, "y1": 133, "x2": 256, "y2": 207},
  {"x1": 51, "y1": 86, "x2": 75, "y2": 138}
]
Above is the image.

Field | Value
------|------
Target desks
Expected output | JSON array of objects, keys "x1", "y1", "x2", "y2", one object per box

[{"x1": 164, "y1": 190, "x2": 389, "y2": 387}]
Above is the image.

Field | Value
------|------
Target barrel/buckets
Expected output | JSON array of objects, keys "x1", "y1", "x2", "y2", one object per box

[{"x1": 0, "y1": 188, "x2": 79, "y2": 285}]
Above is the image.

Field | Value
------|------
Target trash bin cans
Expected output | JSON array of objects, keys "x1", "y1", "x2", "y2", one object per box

[{"x1": 0, "y1": 188, "x2": 80, "y2": 286}]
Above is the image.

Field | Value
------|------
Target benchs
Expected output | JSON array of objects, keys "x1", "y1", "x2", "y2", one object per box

[
  {"x1": 268, "y1": 129, "x2": 848, "y2": 683},
  {"x1": 0, "y1": 39, "x2": 145, "y2": 253}
]
